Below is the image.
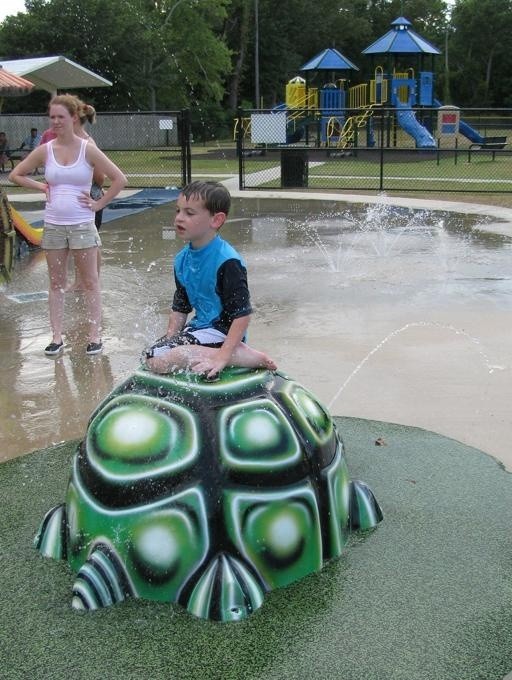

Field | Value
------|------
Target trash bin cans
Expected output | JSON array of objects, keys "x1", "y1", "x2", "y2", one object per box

[{"x1": 281, "y1": 149, "x2": 308, "y2": 188}]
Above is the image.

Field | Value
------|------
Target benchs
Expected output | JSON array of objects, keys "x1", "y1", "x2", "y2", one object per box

[{"x1": 0, "y1": 147, "x2": 41, "y2": 174}]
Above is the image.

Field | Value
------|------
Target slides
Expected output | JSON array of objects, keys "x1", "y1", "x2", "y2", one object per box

[
  {"x1": 395, "y1": 105, "x2": 437, "y2": 148},
  {"x1": 7, "y1": 200, "x2": 45, "y2": 245},
  {"x1": 434, "y1": 98, "x2": 485, "y2": 144}
]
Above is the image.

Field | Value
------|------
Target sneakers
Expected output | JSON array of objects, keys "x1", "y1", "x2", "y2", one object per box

[
  {"x1": 85, "y1": 339, "x2": 103, "y2": 354},
  {"x1": 45, "y1": 337, "x2": 64, "y2": 355}
]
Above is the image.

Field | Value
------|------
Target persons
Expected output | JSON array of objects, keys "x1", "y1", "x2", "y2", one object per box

[
  {"x1": 140, "y1": 181, "x2": 277, "y2": 380},
  {"x1": 65, "y1": 104, "x2": 106, "y2": 293},
  {"x1": 8, "y1": 92, "x2": 130, "y2": 356},
  {"x1": 0, "y1": 132, "x2": 9, "y2": 158},
  {"x1": 18, "y1": 127, "x2": 41, "y2": 175},
  {"x1": 39, "y1": 128, "x2": 57, "y2": 146}
]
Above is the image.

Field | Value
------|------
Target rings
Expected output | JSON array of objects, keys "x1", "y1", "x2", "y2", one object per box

[{"x1": 89, "y1": 203, "x2": 92, "y2": 208}]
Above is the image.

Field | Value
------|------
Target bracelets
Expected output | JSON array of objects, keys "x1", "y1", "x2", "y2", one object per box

[{"x1": 43, "y1": 184, "x2": 48, "y2": 193}]
